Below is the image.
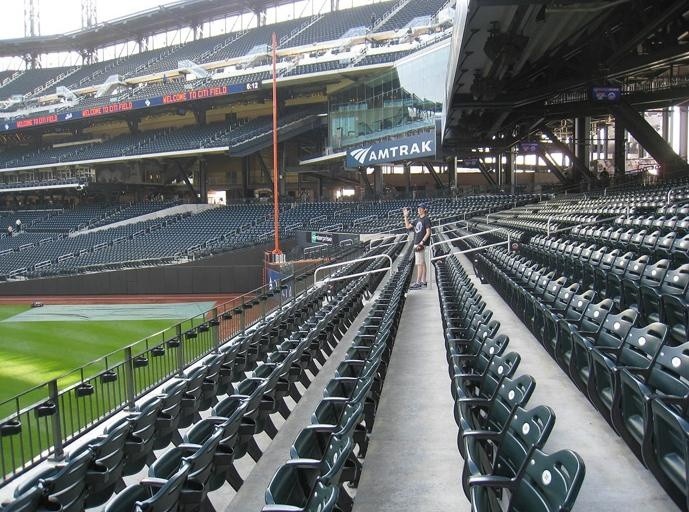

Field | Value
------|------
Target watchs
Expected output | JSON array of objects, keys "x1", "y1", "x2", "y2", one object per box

[{"x1": 422, "y1": 240, "x2": 425, "y2": 243}]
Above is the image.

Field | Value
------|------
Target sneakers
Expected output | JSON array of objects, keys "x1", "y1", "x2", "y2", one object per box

[
  {"x1": 410, "y1": 283, "x2": 421, "y2": 290},
  {"x1": 420, "y1": 282, "x2": 427, "y2": 287}
]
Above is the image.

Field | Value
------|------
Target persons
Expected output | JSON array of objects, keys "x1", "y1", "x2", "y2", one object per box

[
  {"x1": 402, "y1": 202, "x2": 432, "y2": 290},
  {"x1": 8, "y1": 225, "x2": 13, "y2": 235},
  {"x1": 411, "y1": 183, "x2": 418, "y2": 200},
  {"x1": 370, "y1": 12, "x2": 376, "y2": 29},
  {"x1": 406, "y1": 26, "x2": 412, "y2": 35},
  {"x1": 215, "y1": 47, "x2": 343, "y2": 73},
  {"x1": 394, "y1": 38, "x2": 400, "y2": 44},
  {"x1": 16, "y1": 218, "x2": 21, "y2": 231},
  {"x1": 389, "y1": 38, "x2": 395, "y2": 44},
  {"x1": 162, "y1": 74, "x2": 166, "y2": 84},
  {"x1": 599, "y1": 167, "x2": 609, "y2": 178}
]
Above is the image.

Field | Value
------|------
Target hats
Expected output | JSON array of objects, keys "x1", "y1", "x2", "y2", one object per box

[{"x1": 417, "y1": 202, "x2": 427, "y2": 208}]
